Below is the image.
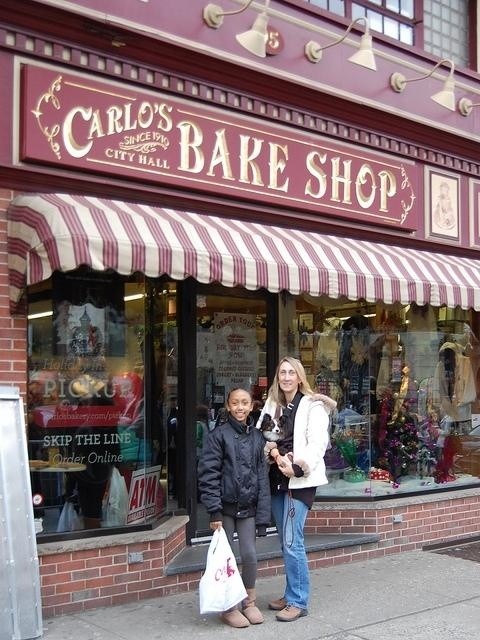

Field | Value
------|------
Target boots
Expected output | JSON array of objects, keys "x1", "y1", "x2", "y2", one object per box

[
  {"x1": 220, "y1": 603, "x2": 250, "y2": 628},
  {"x1": 242, "y1": 588, "x2": 264, "y2": 624}
]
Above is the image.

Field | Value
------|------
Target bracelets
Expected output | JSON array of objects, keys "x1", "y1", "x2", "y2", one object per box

[{"x1": 274, "y1": 453, "x2": 281, "y2": 461}]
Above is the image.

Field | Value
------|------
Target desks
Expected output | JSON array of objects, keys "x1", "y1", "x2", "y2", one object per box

[{"x1": 27, "y1": 461, "x2": 87, "y2": 514}]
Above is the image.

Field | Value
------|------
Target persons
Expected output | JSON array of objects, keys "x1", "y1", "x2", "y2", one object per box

[
  {"x1": 254, "y1": 357, "x2": 330, "y2": 621},
  {"x1": 197, "y1": 387, "x2": 272, "y2": 629}
]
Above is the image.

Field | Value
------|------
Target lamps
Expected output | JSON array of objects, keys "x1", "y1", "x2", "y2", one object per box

[{"x1": 203, "y1": 0, "x2": 456, "y2": 114}]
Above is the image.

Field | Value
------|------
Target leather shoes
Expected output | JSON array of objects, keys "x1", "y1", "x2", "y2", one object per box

[
  {"x1": 276, "y1": 605, "x2": 308, "y2": 622},
  {"x1": 269, "y1": 598, "x2": 287, "y2": 610}
]
